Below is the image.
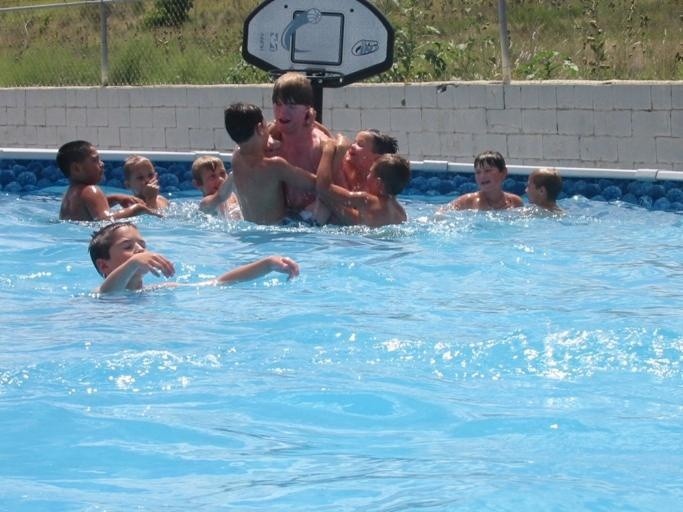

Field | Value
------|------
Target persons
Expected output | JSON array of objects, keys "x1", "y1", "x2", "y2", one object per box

[
  {"x1": 85, "y1": 221, "x2": 299, "y2": 304},
  {"x1": 123, "y1": 154, "x2": 171, "y2": 210},
  {"x1": 55, "y1": 140, "x2": 162, "y2": 223},
  {"x1": 191, "y1": 71, "x2": 409, "y2": 241},
  {"x1": 519, "y1": 167, "x2": 567, "y2": 220},
  {"x1": 438, "y1": 149, "x2": 523, "y2": 216}
]
